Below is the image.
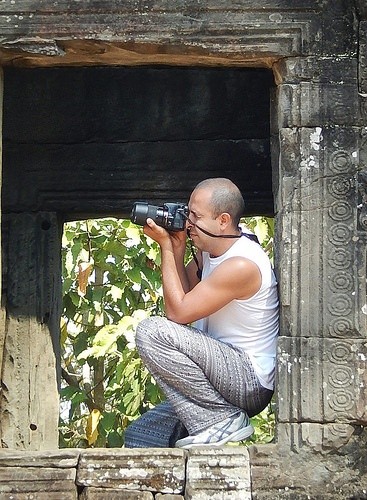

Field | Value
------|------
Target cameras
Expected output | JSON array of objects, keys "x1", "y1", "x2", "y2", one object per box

[{"x1": 130, "y1": 201, "x2": 188, "y2": 232}]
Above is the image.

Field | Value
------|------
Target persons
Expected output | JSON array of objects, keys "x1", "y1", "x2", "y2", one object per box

[{"x1": 122, "y1": 178, "x2": 279, "y2": 448}]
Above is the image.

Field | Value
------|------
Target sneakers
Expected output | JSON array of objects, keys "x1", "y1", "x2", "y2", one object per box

[{"x1": 175, "y1": 408, "x2": 255, "y2": 449}]
